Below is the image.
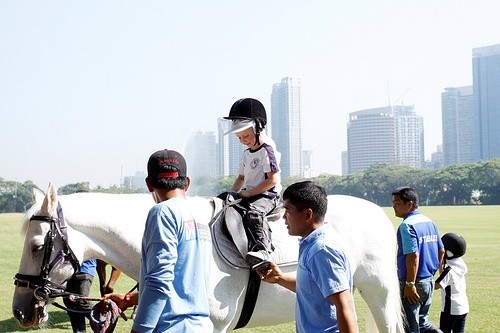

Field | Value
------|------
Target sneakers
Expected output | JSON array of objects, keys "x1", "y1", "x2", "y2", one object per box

[{"x1": 245, "y1": 251, "x2": 275, "y2": 265}]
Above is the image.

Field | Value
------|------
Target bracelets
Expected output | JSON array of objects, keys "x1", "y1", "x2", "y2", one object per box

[{"x1": 124, "y1": 291, "x2": 133, "y2": 308}]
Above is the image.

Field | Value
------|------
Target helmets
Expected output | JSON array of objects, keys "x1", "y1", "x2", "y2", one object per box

[
  {"x1": 441, "y1": 233, "x2": 466, "y2": 259},
  {"x1": 223, "y1": 98, "x2": 266, "y2": 124}
]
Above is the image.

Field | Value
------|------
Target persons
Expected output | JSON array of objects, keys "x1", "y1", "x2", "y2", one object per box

[
  {"x1": 392, "y1": 186, "x2": 445, "y2": 333},
  {"x1": 434, "y1": 232, "x2": 470, "y2": 333},
  {"x1": 222, "y1": 98, "x2": 282, "y2": 273},
  {"x1": 100, "y1": 149, "x2": 215, "y2": 333},
  {"x1": 63, "y1": 258, "x2": 98, "y2": 333},
  {"x1": 255, "y1": 180, "x2": 359, "y2": 333}
]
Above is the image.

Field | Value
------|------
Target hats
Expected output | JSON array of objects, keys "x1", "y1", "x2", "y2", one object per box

[
  {"x1": 148, "y1": 149, "x2": 186, "y2": 179},
  {"x1": 222, "y1": 119, "x2": 262, "y2": 136}
]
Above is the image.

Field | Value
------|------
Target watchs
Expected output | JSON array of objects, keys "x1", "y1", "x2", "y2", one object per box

[{"x1": 406, "y1": 280, "x2": 415, "y2": 287}]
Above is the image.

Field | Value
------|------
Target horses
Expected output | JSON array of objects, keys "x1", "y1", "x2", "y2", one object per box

[{"x1": 12, "y1": 182, "x2": 405, "y2": 333}]
